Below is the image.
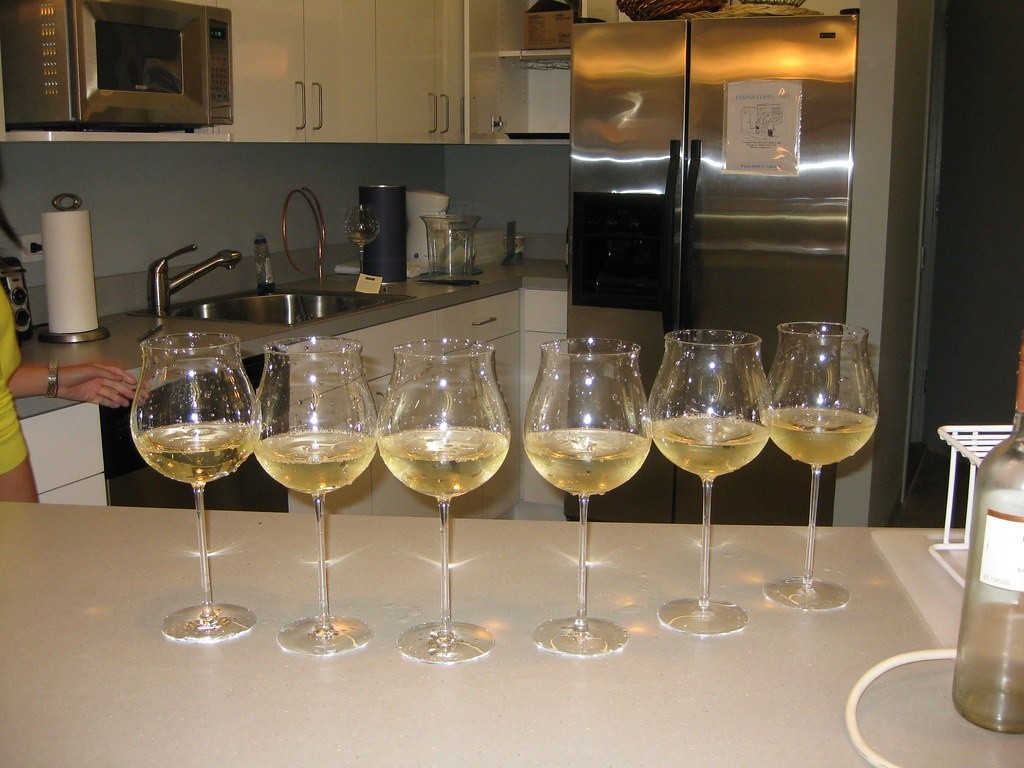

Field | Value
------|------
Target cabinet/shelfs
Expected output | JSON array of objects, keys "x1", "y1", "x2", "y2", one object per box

[
  {"x1": 290, "y1": 289, "x2": 569, "y2": 516},
  {"x1": 16, "y1": 402, "x2": 111, "y2": 506},
  {"x1": 0, "y1": 0, "x2": 621, "y2": 141}
]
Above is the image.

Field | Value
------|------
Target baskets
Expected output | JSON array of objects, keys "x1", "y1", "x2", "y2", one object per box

[
  {"x1": 616, "y1": 0, "x2": 728, "y2": 21},
  {"x1": 739, "y1": 0, "x2": 806, "y2": 7}
]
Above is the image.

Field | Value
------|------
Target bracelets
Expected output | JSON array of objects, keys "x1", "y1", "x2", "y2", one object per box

[{"x1": 44, "y1": 359, "x2": 66, "y2": 405}]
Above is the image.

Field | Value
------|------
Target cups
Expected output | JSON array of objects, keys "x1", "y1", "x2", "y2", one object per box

[{"x1": 419, "y1": 214, "x2": 483, "y2": 282}]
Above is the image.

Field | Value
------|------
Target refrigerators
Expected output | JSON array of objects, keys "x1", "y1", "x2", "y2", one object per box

[{"x1": 564, "y1": 12, "x2": 860, "y2": 527}]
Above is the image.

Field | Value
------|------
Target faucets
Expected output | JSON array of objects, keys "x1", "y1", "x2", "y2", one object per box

[{"x1": 146, "y1": 244, "x2": 242, "y2": 315}]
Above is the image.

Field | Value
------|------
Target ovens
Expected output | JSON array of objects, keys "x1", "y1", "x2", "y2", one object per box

[{"x1": 0, "y1": 0, "x2": 235, "y2": 133}]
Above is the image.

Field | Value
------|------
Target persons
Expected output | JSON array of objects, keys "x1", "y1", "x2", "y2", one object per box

[{"x1": 1, "y1": 155, "x2": 150, "y2": 510}]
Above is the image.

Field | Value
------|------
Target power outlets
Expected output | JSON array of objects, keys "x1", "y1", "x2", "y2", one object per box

[{"x1": 21, "y1": 234, "x2": 43, "y2": 263}]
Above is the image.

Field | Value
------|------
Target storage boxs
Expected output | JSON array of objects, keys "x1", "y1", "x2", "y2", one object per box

[{"x1": 520, "y1": 0, "x2": 576, "y2": 49}]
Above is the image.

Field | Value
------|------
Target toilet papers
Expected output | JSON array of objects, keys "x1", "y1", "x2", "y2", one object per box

[{"x1": 41, "y1": 210, "x2": 99, "y2": 333}]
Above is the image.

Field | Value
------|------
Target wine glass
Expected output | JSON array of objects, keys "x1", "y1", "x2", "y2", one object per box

[
  {"x1": 250, "y1": 336, "x2": 378, "y2": 656},
  {"x1": 522, "y1": 337, "x2": 653, "y2": 658},
  {"x1": 375, "y1": 339, "x2": 512, "y2": 664},
  {"x1": 345, "y1": 204, "x2": 380, "y2": 285},
  {"x1": 655, "y1": 325, "x2": 775, "y2": 636},
  {"x1": 763, "y1": 323, "x2": 881, "y2": 612},
  {"x1": 129, "y1": 330, "x2": 263, "y2": 643}
]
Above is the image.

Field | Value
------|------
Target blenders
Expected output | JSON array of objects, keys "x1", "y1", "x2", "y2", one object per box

[{"x1": 402, "y1": 190, "x2": 450, "y2": 271}]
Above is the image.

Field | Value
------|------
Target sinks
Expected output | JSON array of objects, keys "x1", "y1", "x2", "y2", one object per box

[{"x1": 125, "y1": 287, "x2": 418, "y2": 328}]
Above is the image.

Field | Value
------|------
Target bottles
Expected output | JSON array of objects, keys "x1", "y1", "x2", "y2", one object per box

[
  {"x1": 253, "y1": 232, "x2": 275, "y2": 290},
  {"x1": 952, "y1": 340, "x2": 1024, "y2": 732}
]
